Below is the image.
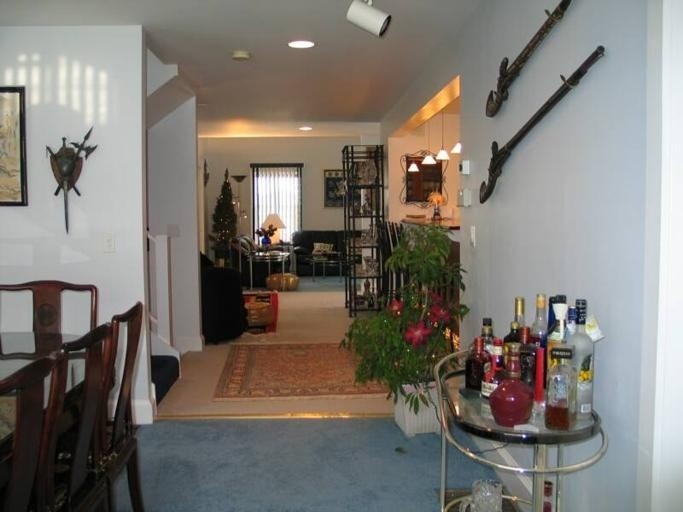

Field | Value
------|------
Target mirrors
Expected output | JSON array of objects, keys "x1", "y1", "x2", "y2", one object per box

[{"x1": 399, "y1": 149, "x2": 449, "y2": 209}]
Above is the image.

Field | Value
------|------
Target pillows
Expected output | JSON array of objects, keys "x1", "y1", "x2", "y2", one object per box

[{"x1": 312, "y1": 242, "x2": 333, "y2": 260}]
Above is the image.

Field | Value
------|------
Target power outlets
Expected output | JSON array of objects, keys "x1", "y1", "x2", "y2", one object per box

[{"x1": 469, "y1": 224, "x2": 476, "y2": 247}]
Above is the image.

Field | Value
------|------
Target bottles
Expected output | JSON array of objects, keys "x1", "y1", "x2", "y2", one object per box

[
  {"x1": 518, "y1": 326, "x2": 535, "y2": 388},
  {"x1": 464, "y1": 337, "x2": 492, "y2": 391},
  {"x1": 544, "y1": 347, "x2": 577, "y2": 431},
  {"x1": 502, "y1": 322, "x2": 523, "y2": 369},
  {"x1": 480, "y1": 338, "x2": 508, "y2": 400},
  {"x1": 487, "y1": 342, "x2": 534, "y2": 428},
  {"x1": 560, "y1": 306, "x2": 576, "y2": 345},
  {"x1": 545, "y1": 295, "x2": 568, "y2": 388},
  {"x1": 528, "y1": 294, "x2": 547, "y2": 347},
  {"x1": 514, "y1": 297, "x2": 525, "y2": 327},
  {"x1": 480, "y1": 318, "x2": 494, "y2": 352},
  {"x1": 539, "y1": 297, "x2": 555, "y2": 389},
  {"x1": 566, "y1": 298, "x2": 594, "y2": 421}
]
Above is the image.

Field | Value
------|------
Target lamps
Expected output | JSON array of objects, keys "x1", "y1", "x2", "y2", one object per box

[
  {"x1": 232, "y1": 174, "x2": 247, "y2": 274},
  {"x1": 428, "y1": 190, "x2": 446, "y2": 221},
  {"x1": 451, "y1": 141, "x2": 461, "y2": 153},
  {"x1": 436, "y1": 111, "x2": 450, "y2": 160},
  {"x1": 421, "y1": 120, "x2": 437, "y2": 164},
  {"x1": 408, "y1": 161, "x2": 420, "y2": 172},
  {"x1": 260, "y1": 214, "x2": 286, "y2": 245},
  {"x1": 346, "y1": 0, "x2": 392, "y2": 39}
]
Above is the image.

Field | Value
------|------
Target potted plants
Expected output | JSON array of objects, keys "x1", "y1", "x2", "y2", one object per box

[
  {"x1": 256, "y1": 224, "x2": 277, "y2": 245},
  {"x1": 211, "y1": 168, "x2": 237, "y2": 267}
]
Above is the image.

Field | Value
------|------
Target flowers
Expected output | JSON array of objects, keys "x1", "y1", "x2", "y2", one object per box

[{"x1": 338, "y1": 293, "x2": 462, "y2": 415}]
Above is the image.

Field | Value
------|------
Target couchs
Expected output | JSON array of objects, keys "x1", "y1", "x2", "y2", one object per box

[
  {"x1": 231, "y1": 234, "x2": 289, "y2": 289},
  {"x1": 292, "y1": 230, "x2": 361, "y2": 277}
]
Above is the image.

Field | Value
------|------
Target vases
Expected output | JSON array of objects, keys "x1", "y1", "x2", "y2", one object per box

[{"x1": 393, "y1": 381, "x2": 441, "y2": 437}]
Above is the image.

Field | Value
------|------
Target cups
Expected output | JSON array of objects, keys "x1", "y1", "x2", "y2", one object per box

[{"x1": 458, "y1": 478, "x2": 502, "y2": 512}]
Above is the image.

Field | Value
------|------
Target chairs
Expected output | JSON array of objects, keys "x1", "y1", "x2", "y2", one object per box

[
  {"x1": 0, "y1": 281, "x2": 97, "y2": 333},
  {"x1": 391, "y1": 222, "x2": 408, "y2": 302},
  {"x1": 35, "y1": 322, "x2": 109, "y2": 512},
  {"x1": 56, "y1": 302, "x2": 158, "y2": 511},
  {"x1": 0, "y1": 357, "x2": 53, "y2": 512},
  {"x1": 379, "y1": 222, "x2": 395, "y2": 308}
]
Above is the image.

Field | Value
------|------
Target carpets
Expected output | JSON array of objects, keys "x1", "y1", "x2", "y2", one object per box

[{"x1": 213, "y1": 343, "x2": 395, "y2": 401}]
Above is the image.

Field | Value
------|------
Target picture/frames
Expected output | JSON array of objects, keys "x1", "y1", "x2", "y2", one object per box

[
  {"x1": 323, "y1": 169, "x2": 345, "y2": 207},
  {"x1": 0, "y1": 86, "x2": 28, "y2": 206}
]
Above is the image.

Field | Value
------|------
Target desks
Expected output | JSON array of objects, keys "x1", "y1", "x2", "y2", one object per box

[{"x1": 432, "y1": 349, "x2": 610, "y2": 511}]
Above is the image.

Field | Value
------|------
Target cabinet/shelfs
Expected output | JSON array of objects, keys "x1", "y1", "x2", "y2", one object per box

[{"x1": 341, "y1": 143, "x2": 385, "y2": 317}]
